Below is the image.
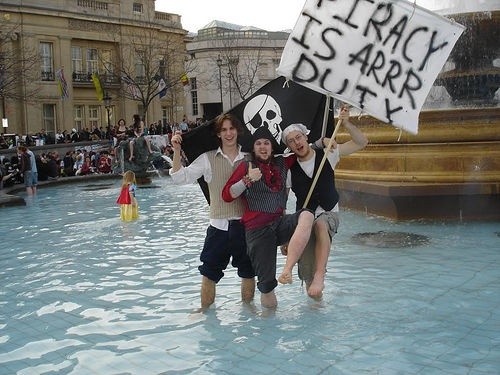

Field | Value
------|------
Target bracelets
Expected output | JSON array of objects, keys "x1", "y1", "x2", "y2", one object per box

[
  {"x1": 243, "y1": 176, "x2": 252, "y2": 188},
  {"x1": 321, "y1": 136, "x2": 327, "y2": 148},
  {"x1": 245, "y1": 173, "x2": 255, "y2": 185}
]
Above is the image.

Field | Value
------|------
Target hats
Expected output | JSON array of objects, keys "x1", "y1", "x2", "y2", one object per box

[{"x1": 252, "y1": 126, "x2": 275, "y2": 146}]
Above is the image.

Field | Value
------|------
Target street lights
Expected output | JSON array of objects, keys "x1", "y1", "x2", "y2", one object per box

[
  {"x1": 216, "y1": 54, "x2": 223, "y2": 113},
  {"x1": 102, "y1": 92, "x2": 113, "y2": 140},
  {"x1": 228, "y1": 62, "x2": 232, "y2": 109}
]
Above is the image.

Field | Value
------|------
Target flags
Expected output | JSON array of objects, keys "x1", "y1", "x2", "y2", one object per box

[
  {"x1": 176, "y1": 74, "x2": 335, "y2": 207},
  {"x1": 55, "y1": 67, "x2": 190, "y2": 106}
]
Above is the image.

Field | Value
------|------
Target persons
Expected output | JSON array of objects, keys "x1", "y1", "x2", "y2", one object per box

[
  {"x1": 0, "y1": 112, "x2": 223, "y2": 187},
  {"x1": 275, "y1": 104, "x2": 368, "y2": 304},
  {"x1": 168, "y1": 113, "x2": 257, "y2": 314},
  {"x1": 116, "y1": 170, "x2": 139, "y2": 222},
  {"x1": 222, "y1": 124, "x2": 338, "y2": 311},
  {"x1": 24, "y1": 146, "x2": 39, "y2": 195},
  {"x1": 18, "y1": 146, "x2": 34, "y2": 197}
]
